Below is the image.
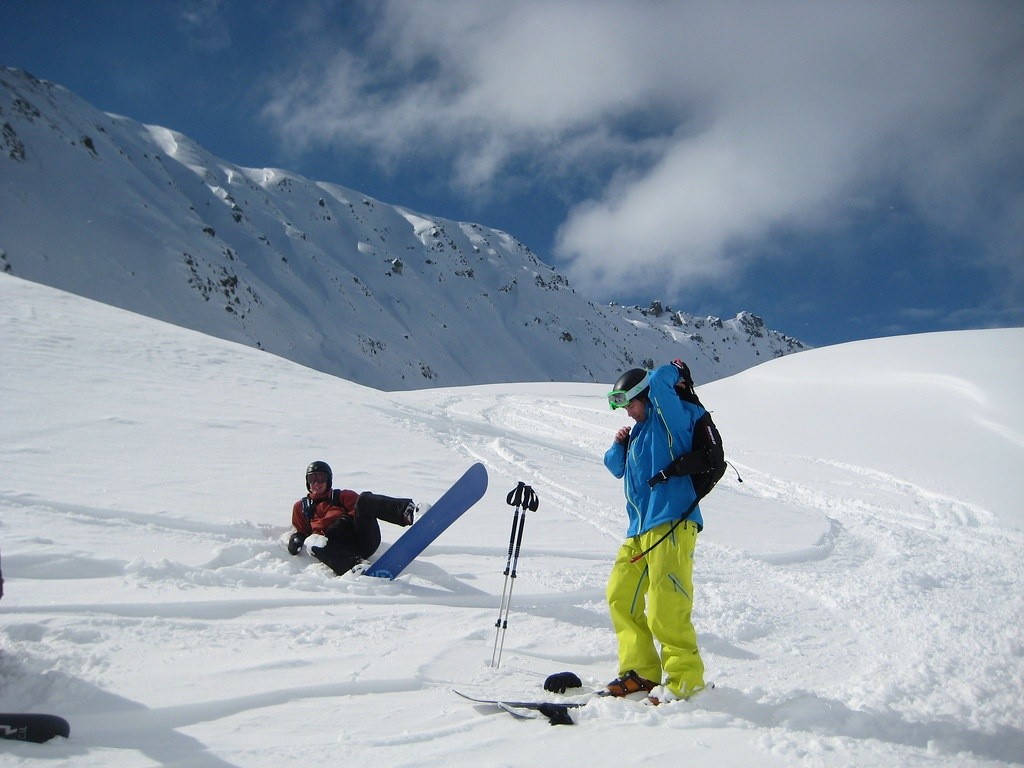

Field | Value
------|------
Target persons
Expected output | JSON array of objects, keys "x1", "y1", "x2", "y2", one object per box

[
  {"x1": 604, "y1": 359, "x2": 704, "y2": 705},
  {"x1": 288, "y1": 461, "x2": 415, "y2": 577}
]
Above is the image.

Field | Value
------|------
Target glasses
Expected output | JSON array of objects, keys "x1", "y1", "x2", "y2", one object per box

[
  {"x1": 608, "y1": 391, "x2": 628, "y2": 409},
  {"x1": 307, "y1": 474, "x2": 330, "y2": 484}
]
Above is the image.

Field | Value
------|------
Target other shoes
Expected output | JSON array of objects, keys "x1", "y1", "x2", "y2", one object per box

[
  {"x1": 604, "y1": 671, "x2": 657, "y2": 695},
  {"x1": 407, "y1": 501, "x2": 429, "y2": 525},
  {"x1": 351, "y1": 558, "x2": 371, "y2": 575}
]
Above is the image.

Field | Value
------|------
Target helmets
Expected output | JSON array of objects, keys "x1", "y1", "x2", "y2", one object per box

[
  {"x1": 613, "y1": 368, "x2": 649, "y2": 403},
  {"x1": 306, "y1": 461, "x2": 332, "y2": 493}
]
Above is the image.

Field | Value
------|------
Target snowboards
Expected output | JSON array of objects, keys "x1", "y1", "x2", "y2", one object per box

[{"x1": 363, "y1": 463, "x2": 489, "y2": 580}]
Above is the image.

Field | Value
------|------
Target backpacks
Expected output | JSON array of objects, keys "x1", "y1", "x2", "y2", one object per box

[{"x1": 647, "y1": 359, "x2": 727, "y2": 503}]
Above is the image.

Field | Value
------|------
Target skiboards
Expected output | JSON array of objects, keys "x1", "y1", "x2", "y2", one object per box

[{"x1": 451, "y1": 688, "x2": 589, "y2": 721}]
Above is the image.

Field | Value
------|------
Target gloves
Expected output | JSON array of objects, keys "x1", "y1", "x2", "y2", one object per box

[
  {"x1": 544, "y1": 672, "x2": 583, "y2": 693},
  {"x1": 288, "y1": 533, "x2": 305, "y2": 555},
  {"x1": 537, "y1": 703, "x2": 574, "y2": 725},
  {"x1": 325, "y1": 515, "x2": 352, "y2": 538}
]
